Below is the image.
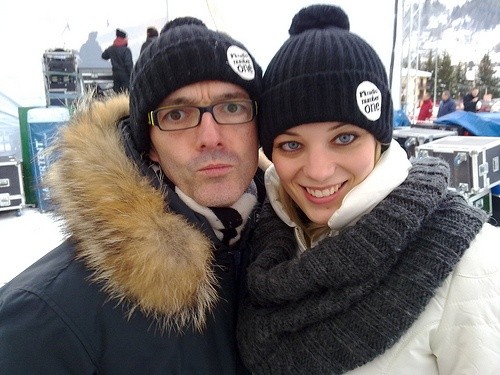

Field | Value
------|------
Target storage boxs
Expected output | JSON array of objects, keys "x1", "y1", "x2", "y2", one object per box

[
  {"x1": 392, "y1": 126, "x2": 500, "y2": 217},
  {"x1": 0, "y1": 155, "x2": 26, "y2": 212}
]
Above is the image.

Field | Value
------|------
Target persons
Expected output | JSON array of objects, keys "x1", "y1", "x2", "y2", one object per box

[
  {"x1": 101, "y1": 30, "x2": 133, "y2": 94},
  {"x1": 418, "y1": 92, "x2": 433, "y2": 121},
  {"x1": 221, "y1": 4, "x2": 500, "y2": 375},
  {"x1": 463, "y1": 87, "x2": 480, "y2": 112},
  {"x1": 437, "y1": 90, "x2": 456, "y2": 117},
  {"x1": 0, "y1": 16, "x2": 268, "y2": 375},
  {"x1": 140, "y1": 28, "x2": 159, "y2": 54}
]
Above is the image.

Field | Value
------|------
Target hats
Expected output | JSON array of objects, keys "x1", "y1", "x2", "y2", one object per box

[
  {"x1": 146, "y1": 28, "x2": 158, "y2": 38},
  {"x1": 129, "y1": 17, "x2": 262, "y2": 164},
  {"x1": 254, "y1": 4, "x2": 394, "y2": 163},
  {"x1": 116, "y1": 29, "x2": 127, "y2": 39}
]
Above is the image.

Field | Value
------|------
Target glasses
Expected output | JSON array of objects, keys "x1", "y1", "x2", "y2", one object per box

[{"x1": 147, "y1": 98, "x2": 257, "y2": 132}]
